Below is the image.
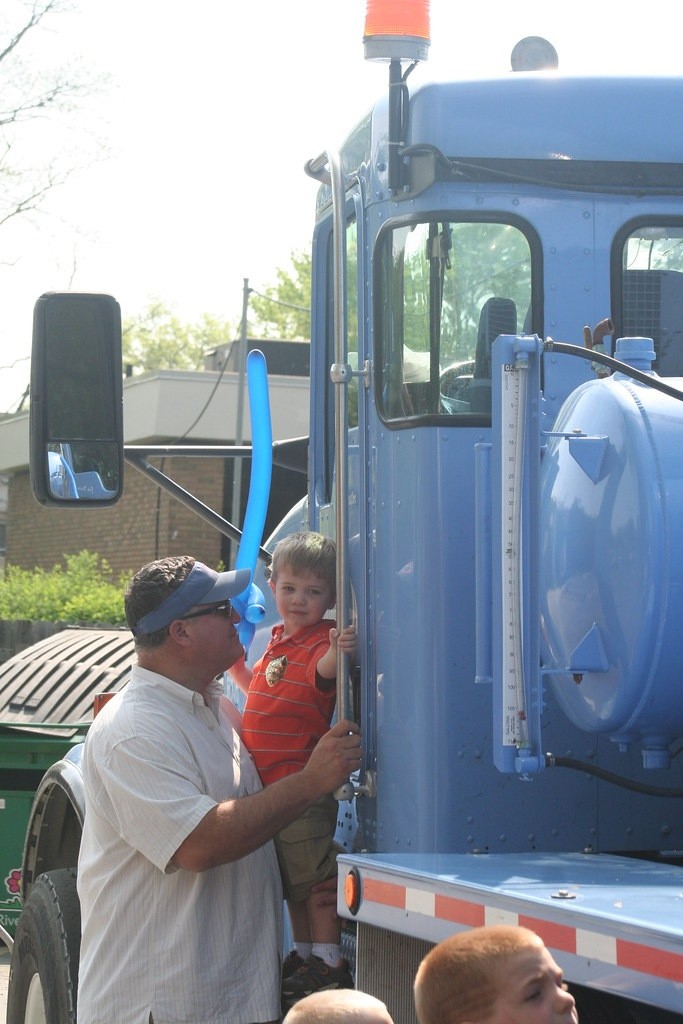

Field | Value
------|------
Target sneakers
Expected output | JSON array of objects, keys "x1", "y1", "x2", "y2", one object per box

[{"x1": 282, "y1": 950, "x2": 356, "y2": 1006}]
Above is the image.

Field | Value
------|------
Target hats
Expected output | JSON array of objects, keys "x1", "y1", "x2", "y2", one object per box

[{"x1": 130, "y1": 561, "x2": 251, "y2": 637}]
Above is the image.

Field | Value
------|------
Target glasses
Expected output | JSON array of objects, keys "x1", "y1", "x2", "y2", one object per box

[{"x1": 180, "y1": 599, "x2": 232, "y2": 621}]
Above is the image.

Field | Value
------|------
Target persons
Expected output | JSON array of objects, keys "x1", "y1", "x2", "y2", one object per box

[
  {"x1": 414, "y1": 920, "x2": 582, "y2": 1024},
  {"x1": 73, "y1": 555, "x2": 362, "y2": 1023},
  {"x1": 223, "y1": 528, "x2": 360, "y2": 996},
  {"x1": 282, "y1": 989, "x2": 399, "y2": 1024}
]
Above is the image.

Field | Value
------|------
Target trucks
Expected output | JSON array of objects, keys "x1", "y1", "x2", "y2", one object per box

[{"x1": 21, "y1": 0, "x2": 683, "y2": 1024}]
